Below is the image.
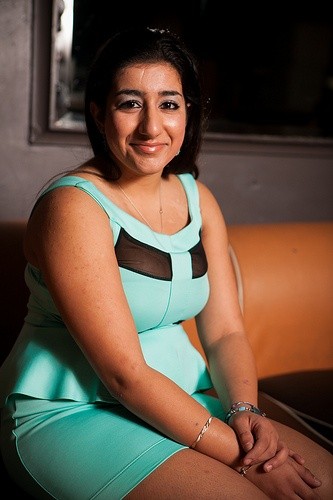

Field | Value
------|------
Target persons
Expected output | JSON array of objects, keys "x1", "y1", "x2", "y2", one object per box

[{"x1": 0, "y1": 25, "x2": 332, "y2": 500}]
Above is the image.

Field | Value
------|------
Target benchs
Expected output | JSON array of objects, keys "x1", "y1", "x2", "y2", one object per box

[{"x1": 0, "y1": 218, "x2": 332, "y2": 500}]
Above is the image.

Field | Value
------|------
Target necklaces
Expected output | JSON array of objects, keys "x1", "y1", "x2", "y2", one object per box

[{"x1": 113, "y1": 174, "x2": 163, "y2": 235}]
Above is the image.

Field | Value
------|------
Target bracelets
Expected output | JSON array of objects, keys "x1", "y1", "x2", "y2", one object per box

[
  {"x1": 189, "y1": 414, "x2": 215, "y2": 451},
  {"x1": 235, "y1": 464, "x2": 254, "y2": 478},
  {"x1": 223, "y1": 400, "x2": 266, "y2": 425}
]
